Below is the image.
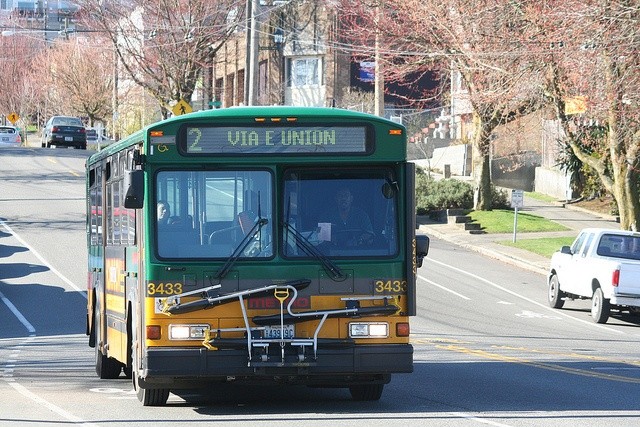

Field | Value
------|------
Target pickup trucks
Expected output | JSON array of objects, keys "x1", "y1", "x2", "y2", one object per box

[{"x1": 547, "y1": 228, "x2": 640, "y2": 323}]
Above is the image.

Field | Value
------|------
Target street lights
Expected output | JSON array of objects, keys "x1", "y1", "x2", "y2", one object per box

[
  {"x1": 248, "y1": 27, "x2": 284, "y2": 106},
  {"x1": 2, "y1": 18, "x2": 62, "y2": 47}
]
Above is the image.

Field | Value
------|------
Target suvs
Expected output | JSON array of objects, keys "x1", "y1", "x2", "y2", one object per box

[{"x1": 86, "y1": 129, "x2": 98, "y2": 140}]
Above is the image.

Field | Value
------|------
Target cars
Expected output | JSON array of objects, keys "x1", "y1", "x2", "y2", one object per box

[
  {"x1": 0, "y1": 125, "x2": 21, "y2": 148},
  {"x1": 41, "y1": 115, "x2": 86, "y2": 149}
]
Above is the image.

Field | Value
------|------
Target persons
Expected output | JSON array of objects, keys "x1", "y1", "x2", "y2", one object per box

[
  {"x1": 156, "y1": 201, "x2": 170, "y2": 232},
  {"x1": 319, "y1": 184, "x2": 375, "y2": 246}
]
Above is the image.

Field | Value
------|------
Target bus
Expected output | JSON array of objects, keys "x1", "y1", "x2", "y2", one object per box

[{"x1": 85, "y1": 106, "x2": 429, "y2": 406}]
